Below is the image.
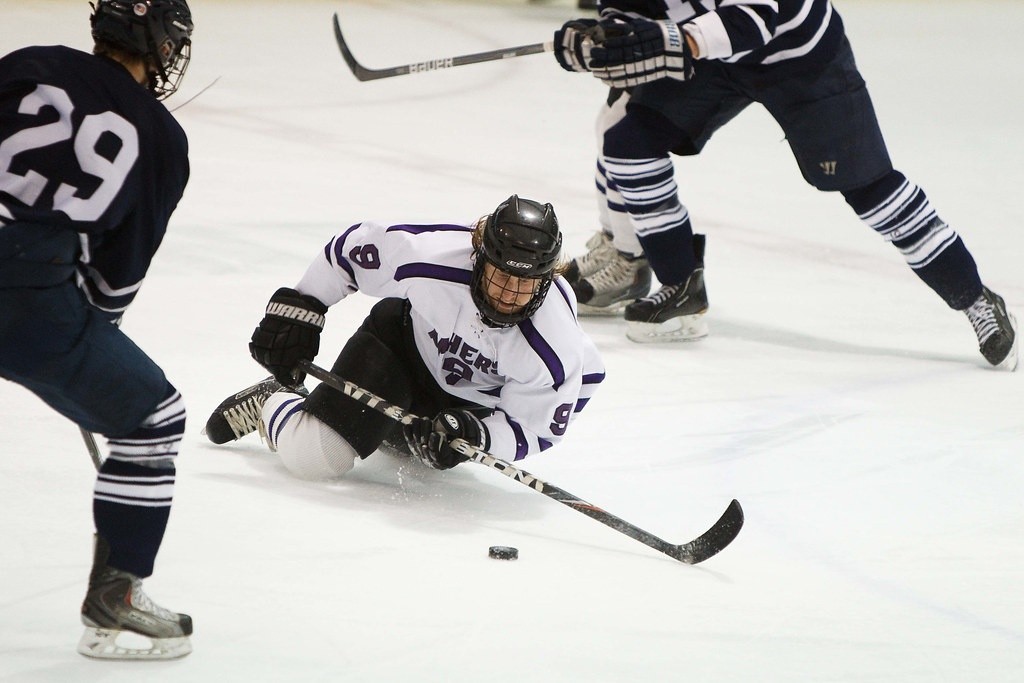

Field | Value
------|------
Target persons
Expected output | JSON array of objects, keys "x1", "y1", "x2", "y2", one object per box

[
  {"x1": 553, "y1": 0, "x2": 1018, "y2": 373},
  {"x1": 201, "y1": 194, "x2": 605, "y2": 481},
  {"x1": 0, "y1": 0, "x2": 193, "y2": 659}
]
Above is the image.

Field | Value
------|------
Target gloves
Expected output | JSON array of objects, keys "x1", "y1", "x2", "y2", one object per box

[
  {"x1": 247, "y1": 287, "x2": 328, "y2": 386},
  {"x1": 589, "y1": 6, "x2": 692, "y2": 90},
  {"x1": 403, "y1": 409, "x2": 488, "y2": 471},
  {"x1": 553, "y1": 17, "x2": 593, "y2": 72}
]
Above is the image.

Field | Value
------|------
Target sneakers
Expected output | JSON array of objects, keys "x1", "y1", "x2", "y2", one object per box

[
  {"x1": 206, "y1": 374, "x2": 310, "y2": 445},
  {"x1": 570, "y1": 251, "x2": 653, "y2": 317},
  {"x1": 562, "y1": 229, "x2": 615, "y2": 285},
  {"x1": 965, "y1": 287, "x2": 1018, "y2": 370},
  {"x1": 77, "y1": 540, "x2": 193, "y2": 662},
  {"x1": 624, "y1": 267, "x2": 711, "y2": 343}
]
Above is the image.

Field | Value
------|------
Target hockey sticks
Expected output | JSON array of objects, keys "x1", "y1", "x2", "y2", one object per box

[
  {"x1": 75, "y1": 421, "x2": 107, "y2": 473},
  {"x1": 295, "y1": 354, "x2": 747, "y2": 566},
  {"x1": 332, "y1": 10, "x2": 558, "y2": 84}
]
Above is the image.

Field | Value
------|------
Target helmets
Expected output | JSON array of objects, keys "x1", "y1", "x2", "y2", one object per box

[
  {"x1": 470, "y1": 195, "x2": 562, "y2": 329},
  {"x1": 90, "y1": 1, "x2": 195, "y2": 101}
]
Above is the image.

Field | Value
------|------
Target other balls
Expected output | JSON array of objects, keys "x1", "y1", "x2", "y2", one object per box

[{"x1": 486, "y1": 545, "x2": 520, "y2": 562}]
